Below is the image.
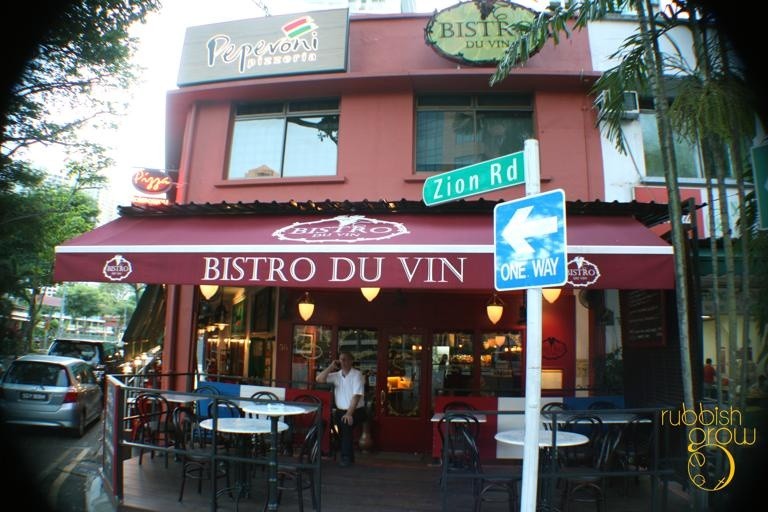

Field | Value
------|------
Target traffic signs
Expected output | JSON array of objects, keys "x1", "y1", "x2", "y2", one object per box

[
  {"x1": 416, "y1": 151, "x2": 530, "y2": 205},
  {"x1": 494, "y1": 187, "x2": 569, "y2": 293}
]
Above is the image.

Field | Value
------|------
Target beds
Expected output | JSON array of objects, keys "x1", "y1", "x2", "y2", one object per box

[{"x1": 431, "y1": 410, "x2": 487, "y2": 476}]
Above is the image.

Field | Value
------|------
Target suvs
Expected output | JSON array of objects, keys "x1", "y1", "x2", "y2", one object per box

[{"x1": 46, "y1": 337, "x2": 121, "y2": 391}]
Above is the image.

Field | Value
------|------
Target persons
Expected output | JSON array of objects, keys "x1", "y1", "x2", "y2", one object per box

[
  {"x1": 315, "y1": 351, "x2": 366, "y2": 468},
  {"x1": 435, "y1": 354, "x2": 447, "y2": 393},
  {"x1": 703, "y1": 358, "x2": 717, "y2": 399}
]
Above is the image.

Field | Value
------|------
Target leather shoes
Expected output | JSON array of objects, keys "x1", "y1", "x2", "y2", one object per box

[{"x1": 343, "y1": 457, "x2": 354, "y2": 464}]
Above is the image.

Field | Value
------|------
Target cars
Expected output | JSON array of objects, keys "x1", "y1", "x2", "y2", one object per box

[{"x1": 0, "y1": 353, "x2": 105, "y2": 440}]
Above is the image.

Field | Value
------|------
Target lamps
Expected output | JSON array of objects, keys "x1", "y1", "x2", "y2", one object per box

[
  {"x1": 360, "y1": 287, "x2": 380, "y2": 302},
  {"x1": 296, "y1": 289, "x2": 316, "y2": 322},
  {"x1": 485, "y1": 291, "x2": 506, "y2": 327},
  {"x1": 542, "y1": 288, "x2": 562, "y2": 304},
  {"x1": 196, "y1": 284, "x2": 228, "y2": 334}
]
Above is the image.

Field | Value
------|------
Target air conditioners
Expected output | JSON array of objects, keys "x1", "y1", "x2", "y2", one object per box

[{"x1": 601, "y1": 87, "x2": 640, "y2": 120}]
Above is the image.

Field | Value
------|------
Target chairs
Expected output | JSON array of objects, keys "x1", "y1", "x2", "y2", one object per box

[
  {"x1": 439, "y1": 401, "x2": 663, "y2": 511},
  {"x1": 134, "y1": 384, "x2": 328, "y2": 511}
]
